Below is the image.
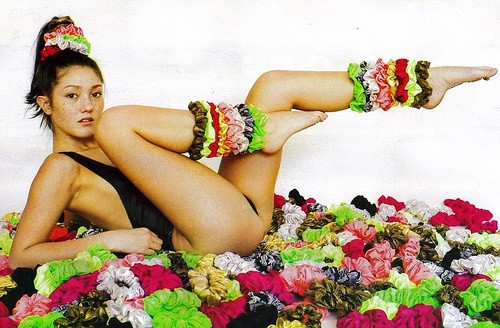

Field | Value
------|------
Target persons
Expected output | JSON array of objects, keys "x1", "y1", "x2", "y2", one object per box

[{"x1": 7, "y1": 15, "x2": 498, "y2": 271}]
